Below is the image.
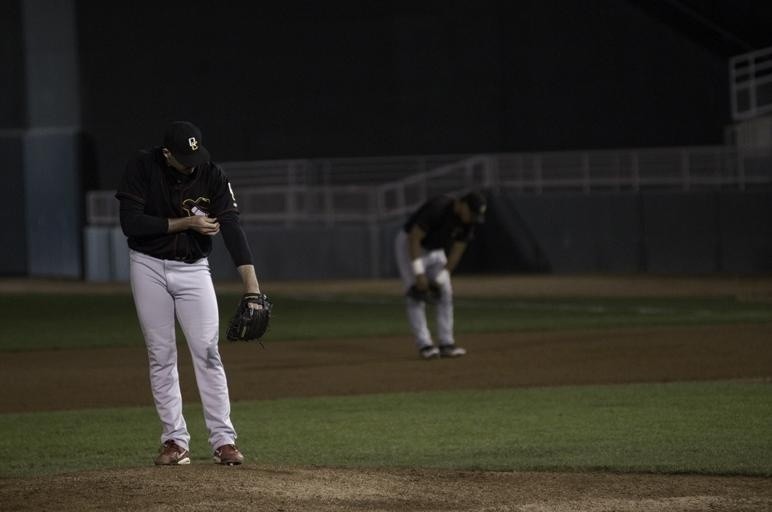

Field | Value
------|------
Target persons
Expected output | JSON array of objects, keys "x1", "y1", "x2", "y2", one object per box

[
  {"x1": 393, "y1": 184, "x2": 490, "y2": 360},
  {"x1": 116, "y1": 117, "x2": 275, "y2": 469}
]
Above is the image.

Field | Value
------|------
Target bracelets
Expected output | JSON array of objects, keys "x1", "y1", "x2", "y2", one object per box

[{"x1": 410, "y1": 257, "x2": 427, "y2": 276}]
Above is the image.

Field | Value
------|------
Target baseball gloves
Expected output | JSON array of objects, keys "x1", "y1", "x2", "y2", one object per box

[
  {"x1": 407, "y1": 280, "x2": 442, "y2": 302},
  {"x1": 226, "y1": 293, "x2": 272, "y2": 342}
]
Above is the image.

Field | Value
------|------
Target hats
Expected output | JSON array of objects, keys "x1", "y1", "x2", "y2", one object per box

[
  {"x1": 164, "y1": 121, "x2": 203, "y2": 168},
  {"x1": 464, "y1": 191, "x2": 488, "y2": 224}
]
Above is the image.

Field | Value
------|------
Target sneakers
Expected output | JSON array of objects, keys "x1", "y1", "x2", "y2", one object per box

[
  {"x1": 213, "y1": 444, "x2": 244, "y2": 464},
  {"x1": 156, "y1": 443, "x2": 191, "y2": 465},
  {"x1": 419, "y1": 345, "x2": 440, "y2": 359},
  {"x1": 441, "y1": 345, "x2": 466, "y2": 357}
]
